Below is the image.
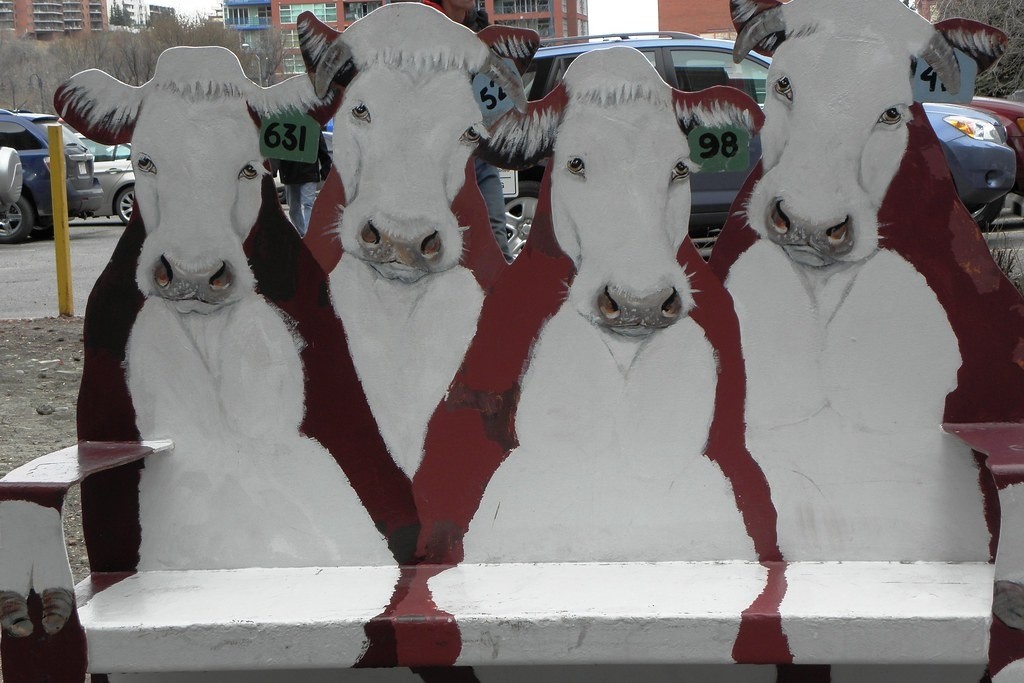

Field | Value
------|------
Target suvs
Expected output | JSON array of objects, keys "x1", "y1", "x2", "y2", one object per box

[
  {"x1": 0, "y1": 146, "x2": 23, "y2": 216},
  {"x1": 0, "y1": 108, "x2": 105, "y2": 244},
  {"x1": 481, "y1": 30, "x2": 1016, "y2": 266}
]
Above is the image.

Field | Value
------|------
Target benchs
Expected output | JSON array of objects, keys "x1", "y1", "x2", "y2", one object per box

[{"x1": 0, "y1": 1, "x2": 1024, "y2": 683}]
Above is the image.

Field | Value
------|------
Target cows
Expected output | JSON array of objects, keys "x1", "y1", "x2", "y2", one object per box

[{"x1": 53, "y1": 0, "x2": 1024, "y2": 683}]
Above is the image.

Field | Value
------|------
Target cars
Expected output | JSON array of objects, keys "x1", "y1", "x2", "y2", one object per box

[
  {"x1": 963, "y1": 96, "x2": 1024, "y2": 232},
  {"x1": 58, "y1": 117, "x2": 135, "y2": 225}
]
